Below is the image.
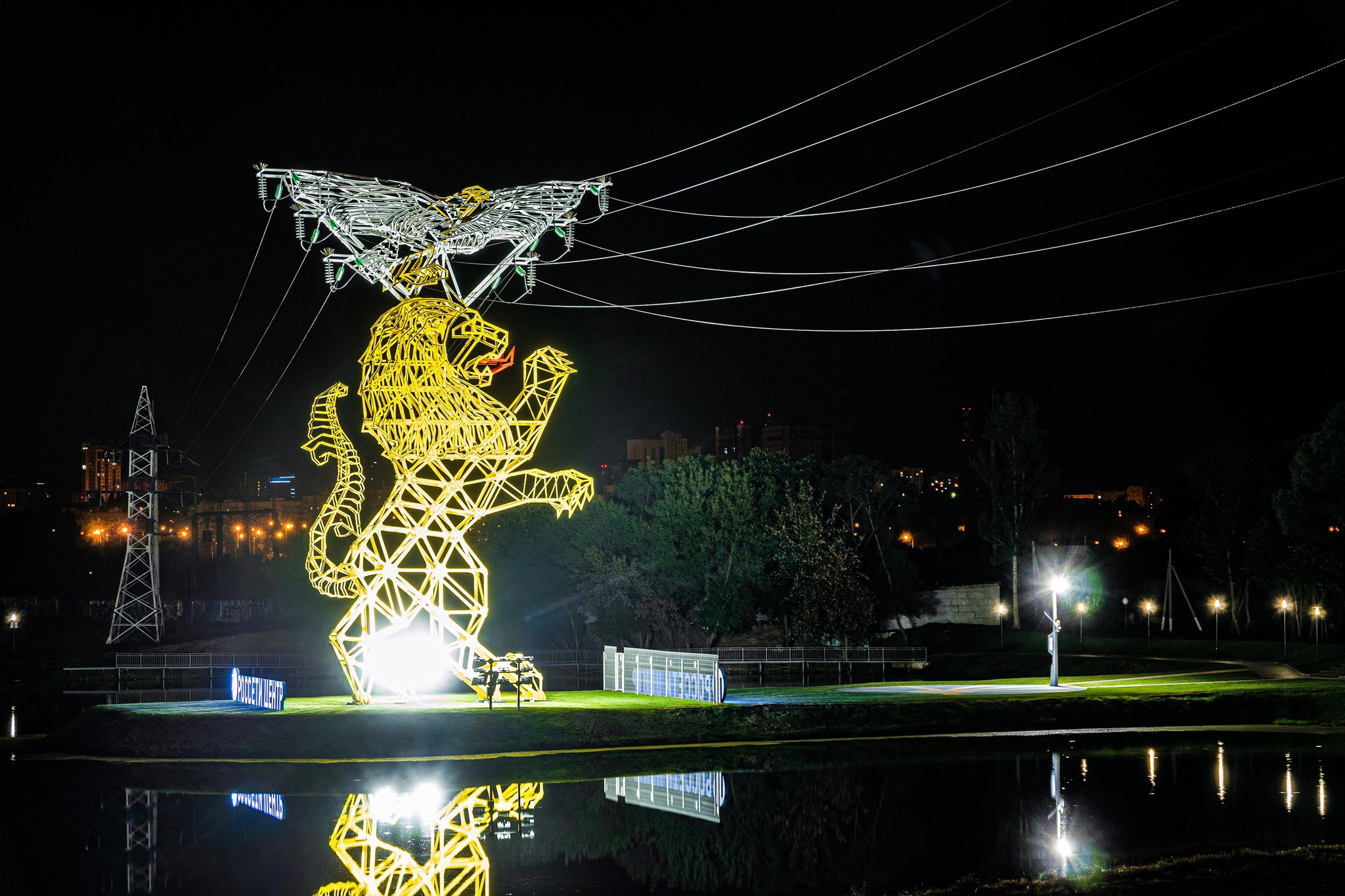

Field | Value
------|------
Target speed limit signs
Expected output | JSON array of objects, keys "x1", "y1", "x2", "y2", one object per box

[{"x1": 1122, "y1": 598, "x2": 1129, "y2": 605}]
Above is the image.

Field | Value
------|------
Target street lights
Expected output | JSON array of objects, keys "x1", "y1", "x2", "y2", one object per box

[
  {"x1": 1047, "y1": 573, "x2": 1066, "y2": 686},
  {"x1": 1312, "y1": 606, "x2": 1322, "y2": 668},
  {"x1": 1078, "y1": 603, "x2": 1084, "y2": 654},
  {"x1": 1213, "y1": 599, "x2": 1220, "y2": 656},
  {"x1": 998, "y1": 605, "x2": 1004, "y2": 653},
  {"x1": 1280, "y1": 600, "x2": 1289, "y2": 663},
  {"x1": 1145, "y1": 602, "x2": 1151, "y2": 657}
]
